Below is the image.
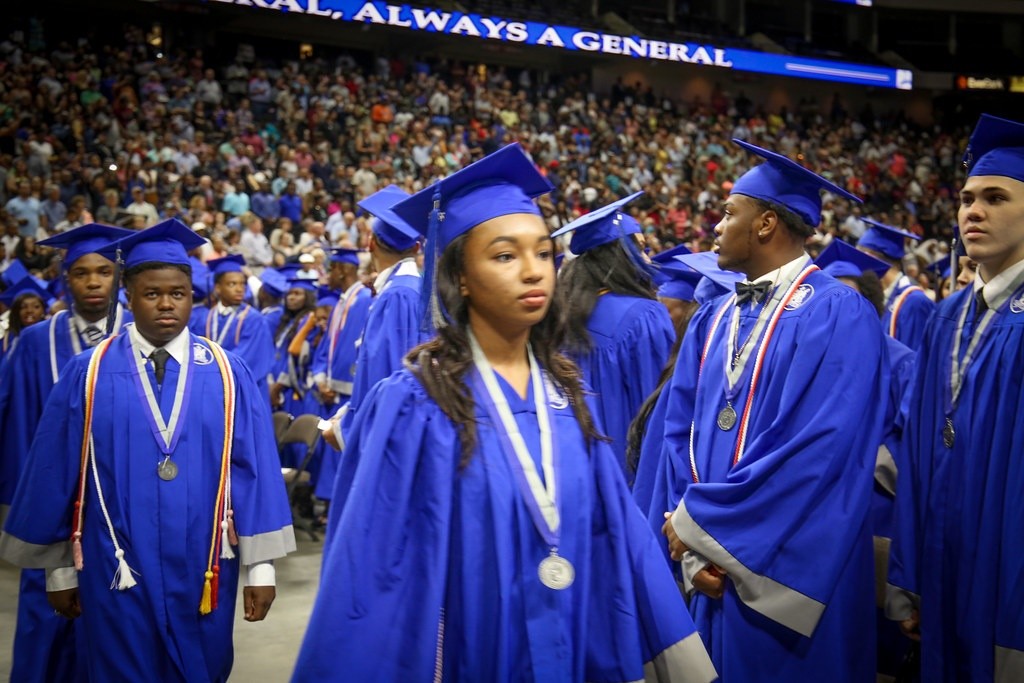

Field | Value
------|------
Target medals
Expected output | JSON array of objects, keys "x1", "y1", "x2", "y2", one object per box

[
  {"x1": 538, "y1": 556, "x2": 574, "y2": 589},
  {"x1": 157, "y1": 459, "x2": 179, "y2": 481},
  {"x1": 717, "y1": 406, "x2": 737, "y2": 431},
  {"x1": 350, "y1": 361, "x2": 359, "y2": 378},
  {"x1": 943, "y1": 425, "x2": 956, "y2": 449}
]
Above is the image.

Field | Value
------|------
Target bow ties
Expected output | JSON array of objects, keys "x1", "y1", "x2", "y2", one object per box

[{"x1": 733, "y1": 281, "x2": 773, "y2": 305}]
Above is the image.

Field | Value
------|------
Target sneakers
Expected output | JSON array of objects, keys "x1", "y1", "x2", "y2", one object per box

[{"x1": 86, "y1": 327, "x2": 104, "y2": 348}]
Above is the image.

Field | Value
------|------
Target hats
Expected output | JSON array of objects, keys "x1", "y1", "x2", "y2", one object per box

[
  {"x1": 33, "y1": 224, "x2": 138, "y2": 270},
  {"x1": 93, "y1": 217, "x2": 208, "y2": 334},
  {"x1": 356, "y1": 183, "x2": 422, "y2": 250},
  {"x1": 650, "y1": 202, "x2": 978, "y2": 313},
  {"x1": 960, "y1": 113, "x2": 1024, "y2": 181},
  {"x1": 550, "y1": 191, "x2": 643, "y2": 255},
  {"x1": 388, "y1": 142, "x2": 558, "y2": 331},
  {"x1": 0, "y1": 247, "x2": 369, "y2": 345},
  {"x1": 729, "y1": 138, "x2": 863, "y2": 227}
]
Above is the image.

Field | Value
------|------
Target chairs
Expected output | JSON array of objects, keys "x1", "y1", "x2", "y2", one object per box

[{"x1": 269, "y1": 410, "x2": 325, "y2": 542}]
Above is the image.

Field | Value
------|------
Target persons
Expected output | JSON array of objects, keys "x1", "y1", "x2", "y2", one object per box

[
  {"x1": 265, "y1": 246, "x2": 373, "y2": 529},
  {"x1": 0, "y1": 224, "x2": 145, "y2": 683},
  {"x1": 886, "y1": 111, "x2": 1024, "y2": 683},
  {"x1": 324, "y1": 182, "x2": 443, "y2": 453},
  {"x1": 643, "y1": 138, "x2": 892, "y2": 683},
  {"x1": 0, "y1": 216, "x2": 297, "y2": 683},
  {"x1": 0, "y1": 23, "x2": 1024, "y2": 345},
  {"x1": 291, "y1": 142, "x2": 717, "y2": 683},
  {"x1": 550, "y1": 190, "x2": 680, "y2": 487}
]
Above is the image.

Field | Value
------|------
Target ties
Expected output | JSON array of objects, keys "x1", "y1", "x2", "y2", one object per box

[
  {"x1": 148, "y1": 350, "x2": 170, "y2": 390},
  {"x1": 969, "y1": 288, "x2": 990, "y2": 338}
]
